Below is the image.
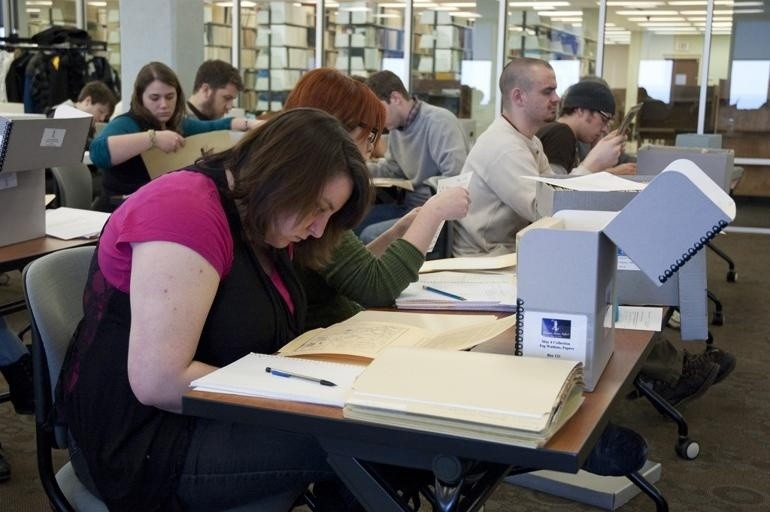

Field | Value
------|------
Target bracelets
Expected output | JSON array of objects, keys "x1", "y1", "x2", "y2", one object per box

[{"x1": 146, "y1": 129, "x2": 156, "y2": 150}]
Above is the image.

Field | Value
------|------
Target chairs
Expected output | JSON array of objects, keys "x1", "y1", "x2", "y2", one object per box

[
  {"x1": 52, "y1": 160, "x2": 105, "y2": 221},
  {"x1": 21, "y1": 243, "x2": 112, "y2": 512}
]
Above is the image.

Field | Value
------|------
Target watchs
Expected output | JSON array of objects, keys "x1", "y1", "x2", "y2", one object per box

[{"x1": 240, "y1": 118, "x2": 248, "y2": 131}]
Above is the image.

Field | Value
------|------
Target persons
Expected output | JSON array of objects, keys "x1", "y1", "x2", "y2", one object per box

[
  {"x1": 44, "y1": 80, "x2": 118, "y2": 210},
  {"x1": 88, "y1": 60, "x2": 268, "y2": 213},
  {"x1": 350, "y1": 69, "x2": 473, "y2": 245},
  {"x1": 577, "y1": 74, "x2": 638, "y2": 166},
  {"x1": 350, "y1": 74, "x2": 387, "y2": 157},
  {"x1": 637, "y1": 87, "x2": 652, "y2": 103},
  {"x1": 452, "y1": 56, "x2": 629, "y2": 260},
  {"x1": 285, "y1": 66, "x2": 475, "y2": 328},
  {"x1": 534, "y1": 80, "x2": 637, "y2": 175},
  {"x1": 182, "y1": 58, "x2": 245, "y2": 120},
  {"x1": 54, "y1": 105, "x2": 374, "y2": 512}
]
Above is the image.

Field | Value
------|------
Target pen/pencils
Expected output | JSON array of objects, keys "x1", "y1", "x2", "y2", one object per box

[
  {"x1": 266, "y1": 367, "x2": 338, "y2": 386},
  {"x1": 423, "y1": 285, "x2": 467, "y2": 300}
]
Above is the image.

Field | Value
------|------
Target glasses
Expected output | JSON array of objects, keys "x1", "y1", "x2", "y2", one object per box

[{"x1": 358, "y1": 121, "x2": 378, "y2": 143}]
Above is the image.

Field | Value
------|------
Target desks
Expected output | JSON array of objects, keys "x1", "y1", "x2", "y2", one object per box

[
  {"x1": 0, "y1": 235, "x2": 97, "y2": 297},
  {"x1": 174, "y1": 159, "x2": 750, "y2": 512}
]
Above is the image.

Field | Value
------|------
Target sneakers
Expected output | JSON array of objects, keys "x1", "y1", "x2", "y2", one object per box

[
  {"x1": 648, "y1": 361, "x2": 721, "y2": 419},
  {"x1": 682, "y1": 349, "x2": 738, "y2": 387},
  {"x1": 0, "y1": 450, "x2": 13, "y2": 483},
  {"x1": 581, "y1": 422, "x2": 650, "y2": 477}
]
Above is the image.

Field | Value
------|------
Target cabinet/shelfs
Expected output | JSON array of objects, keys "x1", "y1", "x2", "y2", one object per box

[{"x1": 26, "y1": 0, "x2": 602, "y2": 123}]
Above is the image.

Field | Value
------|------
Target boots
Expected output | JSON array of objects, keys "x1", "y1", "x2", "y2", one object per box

[{"x1": 0, "y1": 353, "x2": 36, "y2": 419}]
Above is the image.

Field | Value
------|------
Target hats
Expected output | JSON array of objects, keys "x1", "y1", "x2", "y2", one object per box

[{"x1": 562, "y1": 75, "x2": 616, "y2": 115}]
